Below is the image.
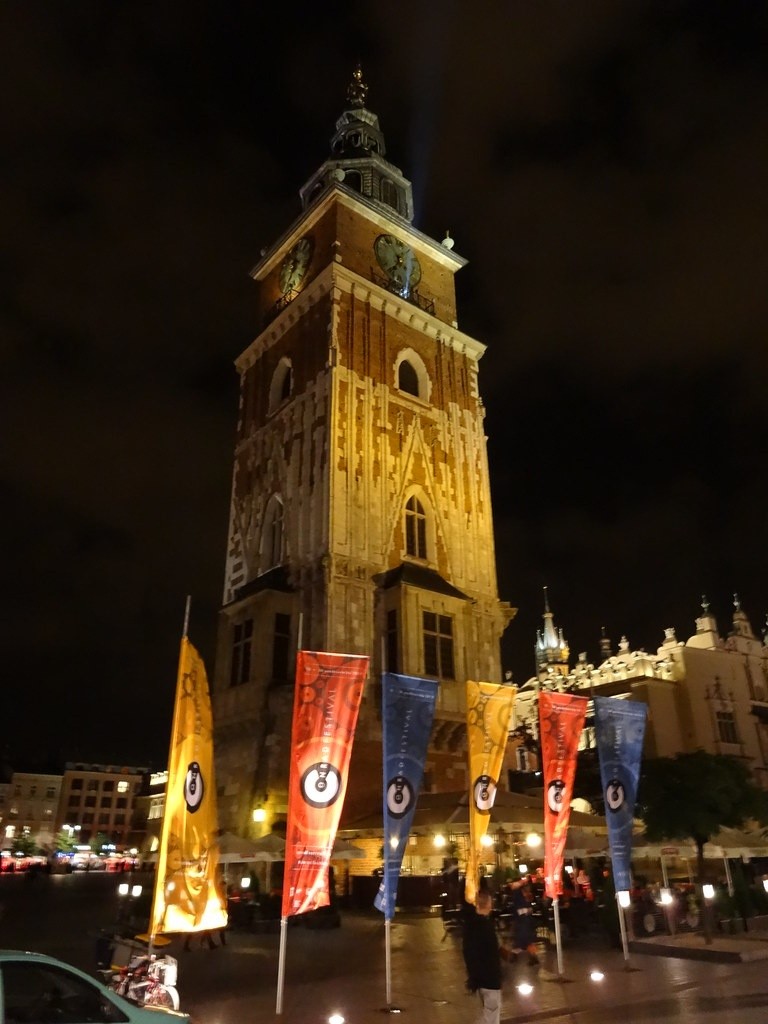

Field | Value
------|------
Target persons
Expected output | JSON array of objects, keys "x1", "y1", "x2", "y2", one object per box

[
  {"x1": 84, "y1": 859, "x2": 137, "y2": 872},
  {"x1": 497, "y1": 862, "x2": 646, "y2": 957},
  {"x1": 220, "y1": 870, "x2": 282, "y2": 931},
  {"x1": 462, "y1": 890, "x2": 504, "y2": 1023}
]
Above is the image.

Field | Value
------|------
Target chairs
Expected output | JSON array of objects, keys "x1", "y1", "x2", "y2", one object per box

[
  {"x1": 434, "y1": 874, "x2": 617, "y2": 949},
  {"x1": 222, "y1": 880, "x2": 292, "y2": 933}
]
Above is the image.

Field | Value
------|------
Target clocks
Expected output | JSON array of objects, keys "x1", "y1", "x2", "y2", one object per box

[
  {"x1": 373, "y1": 233, "x2": 422, "y2": 289},
  {"x1": 277, "y1": 235, "x2": 314, "y2": 293}
]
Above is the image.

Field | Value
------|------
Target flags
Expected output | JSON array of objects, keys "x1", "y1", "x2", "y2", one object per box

[
  {"x1": 586, "y1": 692, "x2": 649, "y2": 890},
  {"x1": 135, "y1": 636, "x2": 233, "y2": 937},
  {"x1": 280, "y1": 648, "x2": 371, "y2": 919},
  {"x1": 374, "y1": 673, "x2": 440, "y2": 912},
  {"x1": 459, "y1": 681, "x2": 520, "y2": 911},
  {"x1": 538, "y1": 686, "x2": 591, "y2": 896}
]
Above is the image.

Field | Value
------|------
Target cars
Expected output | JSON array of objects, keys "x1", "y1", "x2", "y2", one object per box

[{"x1": 0, "y1": 950, "x2": 196, "y2": 1024}]
